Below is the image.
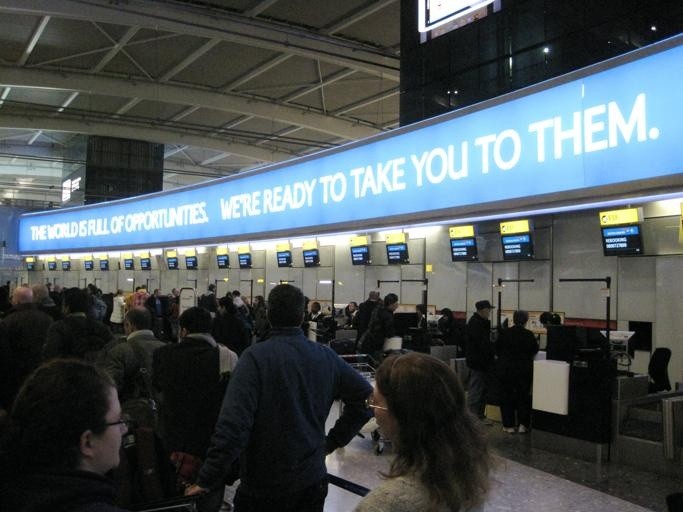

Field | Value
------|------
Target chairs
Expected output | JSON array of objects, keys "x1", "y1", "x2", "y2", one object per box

[{"x1": 646, "y1": 347, "x2": 673, "y2": 388}]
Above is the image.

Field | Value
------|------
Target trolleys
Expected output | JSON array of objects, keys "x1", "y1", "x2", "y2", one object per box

[{"x1": 330, "y1": 351, "x2": 393, "y2": 455}]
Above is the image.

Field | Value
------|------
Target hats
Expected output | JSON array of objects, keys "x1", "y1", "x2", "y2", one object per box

[{"x1": 475, "y1": 300, "x2": 496, "y2": 310}]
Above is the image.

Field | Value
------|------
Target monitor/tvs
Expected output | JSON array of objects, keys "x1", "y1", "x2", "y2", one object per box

[
  {"x1": 601, "y1": 223, "x2": 642, "y2": 255},
  {"x1": 26, "y1": 257, "x2": 200, "y2": 270},
  {"x1": 449, "y1": 238, "x2": 480, "y2": 262},
  {"x1": 598, "y1": 330, "x2": 635, "y2": 359},
  {"x1": 426, "y1": 314, "x2": 443, "y2": 332},
  {"x1": 216, "y1": 245, "x2": 408, "y2": 269},
  {"x1": 335, "y1": 304, "x2": 349, "y2": 317},
  {"x1": 501, "y1": 234, "x2": 534, "y2": 260}
]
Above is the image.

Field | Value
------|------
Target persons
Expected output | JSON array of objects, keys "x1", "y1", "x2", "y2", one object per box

[{"x1": 0, "y1": 274, "x2": 565, "y2": 512}]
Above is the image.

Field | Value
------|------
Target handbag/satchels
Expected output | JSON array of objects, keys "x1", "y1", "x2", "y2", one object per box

[
  {"x1": 122, "y1": 433, "x2": 165, "y2": 459},
  {"x1": 355, "y1": 332, "x2": 373, "y2": 364}
]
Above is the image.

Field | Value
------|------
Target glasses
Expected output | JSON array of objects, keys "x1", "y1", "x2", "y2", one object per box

[
  {"x1": 71, "y1": 413, "x2": 136, "y2": 436},
  {"x1": 363, "y1": 392, "x2": 389, "y2": 411}
]
